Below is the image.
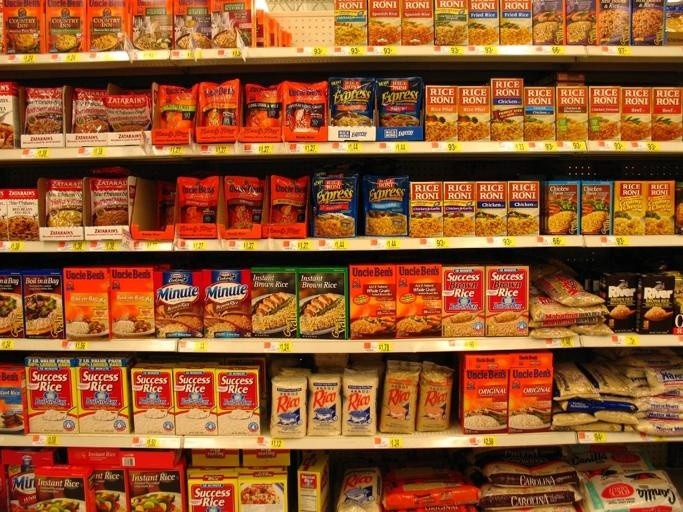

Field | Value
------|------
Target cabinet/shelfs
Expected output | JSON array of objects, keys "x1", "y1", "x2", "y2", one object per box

[{"x1": 0, "y1": 1, "x2": 677, "y2": 507}]
